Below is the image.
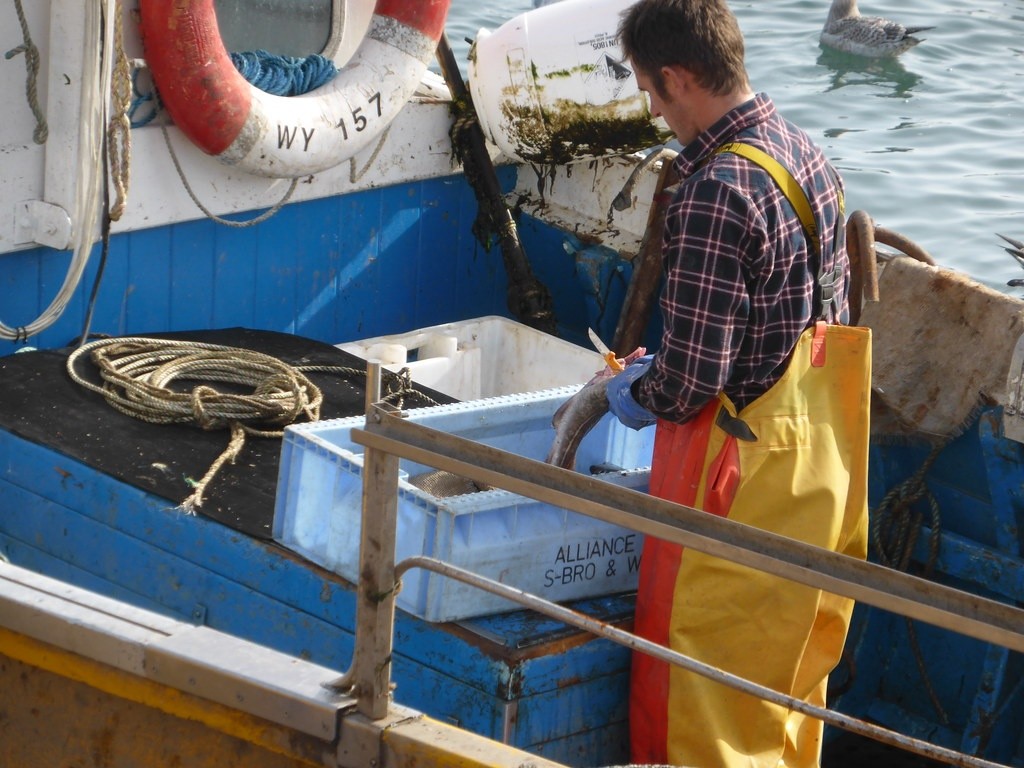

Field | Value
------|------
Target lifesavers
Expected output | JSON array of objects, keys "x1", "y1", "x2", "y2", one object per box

[{"x1": 137, "y1": 0, "x2": 451, "y2": 179}]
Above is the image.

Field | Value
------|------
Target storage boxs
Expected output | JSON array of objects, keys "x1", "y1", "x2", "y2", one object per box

[{"x1": 270, "y1": 316, "x2": 658, "y2": 624}]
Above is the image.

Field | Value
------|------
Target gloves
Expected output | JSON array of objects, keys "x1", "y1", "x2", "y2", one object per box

[{"x1": 606, "y1": 354, "x2": 657, "y2": 431}]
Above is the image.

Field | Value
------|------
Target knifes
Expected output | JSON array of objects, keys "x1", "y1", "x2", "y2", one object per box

[{"x1": 588, "y1": 327, "x2": 624, "y2": 375}]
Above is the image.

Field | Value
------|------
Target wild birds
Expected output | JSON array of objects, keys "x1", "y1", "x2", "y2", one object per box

[
  {"x1": 995, "y1": 232, "x2": 1024, "y2": 271},
  {"x1": 819, "y1": 1, "x2": 937, "y2": 59}
]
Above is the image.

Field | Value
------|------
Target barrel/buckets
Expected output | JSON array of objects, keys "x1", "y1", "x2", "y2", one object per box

[{"x1": 469, "y1": 0, "x2": 676, "y2": 164}]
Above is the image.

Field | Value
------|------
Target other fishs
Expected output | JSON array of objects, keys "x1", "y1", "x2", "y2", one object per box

[{"x1": 546, "y1": 373, "x2": 618, "y2": 472}]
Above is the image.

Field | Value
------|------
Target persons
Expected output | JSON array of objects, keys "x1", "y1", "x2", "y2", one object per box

[{"x1": 602, "y1": 0, "x2": 873, "y2": 768}]
